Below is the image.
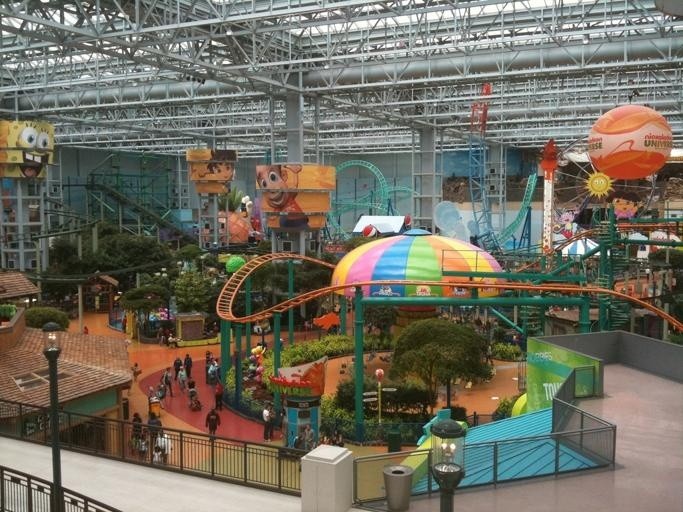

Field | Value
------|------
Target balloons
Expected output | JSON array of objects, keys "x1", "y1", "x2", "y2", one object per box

[{"x1": 587, "y1": 104, "x2": 674, "y2": 180}]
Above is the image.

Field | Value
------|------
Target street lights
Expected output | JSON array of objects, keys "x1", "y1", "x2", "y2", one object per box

[
  {"x1": 425, "y1": 414, "x2": 465, "y2": 511},
  {"x1": 32, "y1": 297, "x2": 37, "y2": 309},
  {"x1": 154, "y1": 240, "x2": 221, "y2": 321},
  {"x1": 39, "y1": 320, "x2": 70, "y2": 510},
  {"x1": 375, "y1": 369, "x2": 384, "y2": 428}
]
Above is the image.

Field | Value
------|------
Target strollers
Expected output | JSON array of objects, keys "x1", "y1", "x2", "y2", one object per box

[{"x1": 187, "y1": 389, "x2": 201, "y2": 411}]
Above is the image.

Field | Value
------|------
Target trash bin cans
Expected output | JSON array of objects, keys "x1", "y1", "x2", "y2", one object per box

[
  {"x1": 382, "y1": 466, "x2": 413, "y2": 512},
  {"x1": 122, "y1": 398, "x2": 129, "y2": 419},
  {"x1": 388, "y1": 433, "x2": 401, "y2": 453}
]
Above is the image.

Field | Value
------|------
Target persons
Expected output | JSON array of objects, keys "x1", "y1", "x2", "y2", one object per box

[
  {"x1": 262, "y1": 402, "x2": 274, "y2": 442},
  {"x1": 485, "y1": 344, "x2": 493, "y2": 366},
  {"x1": 300, "y1": 423, "x2": 343, "y2": 450},
  {"x1": 156, "y1": 326, "x2": 175, "y2": 346},
  {"x1": 204, "y1": 408, "x2": 220, "y2": 440},
  {"x1": 128, "y1": 412, "x2": 172, "y2": 463},
  {"x1": 122, "y1": 315, "x2": 127, "y2": 333},
  {"x1": 148, "y1": 350, "x2": 223, "y2": 411}
]
